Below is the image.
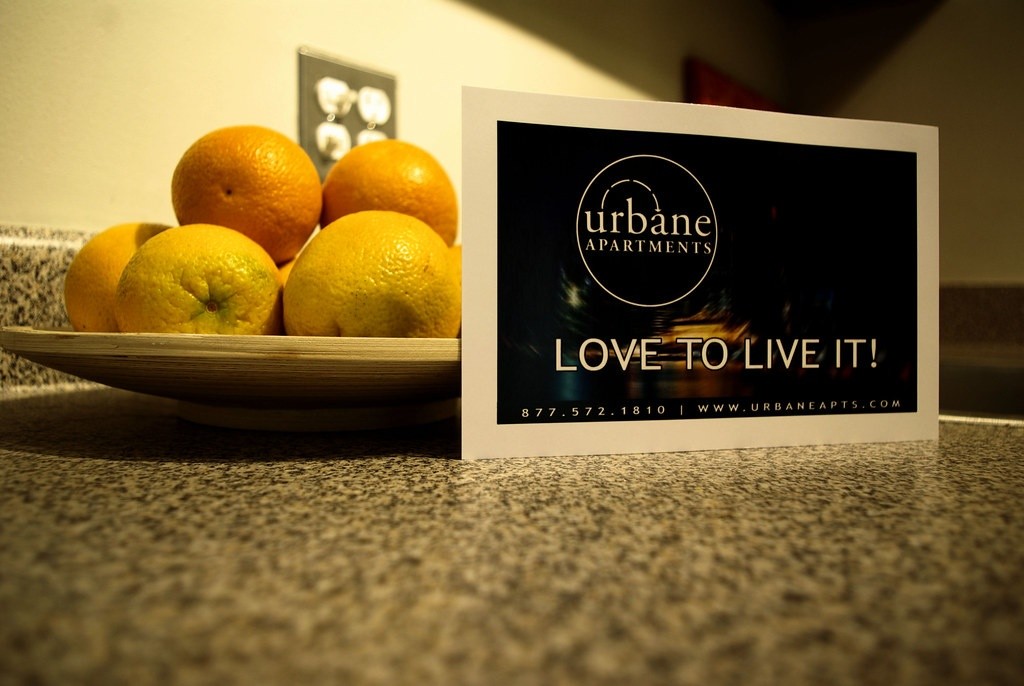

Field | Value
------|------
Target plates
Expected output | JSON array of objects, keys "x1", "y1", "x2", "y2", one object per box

[{"x1": 0, "y1": 324, "x2": 460, "y2": 429}]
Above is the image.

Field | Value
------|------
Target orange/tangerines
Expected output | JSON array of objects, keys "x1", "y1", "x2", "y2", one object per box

[{"x1": 61, "y1": 125, "x2": 462, "y2": 341}]
